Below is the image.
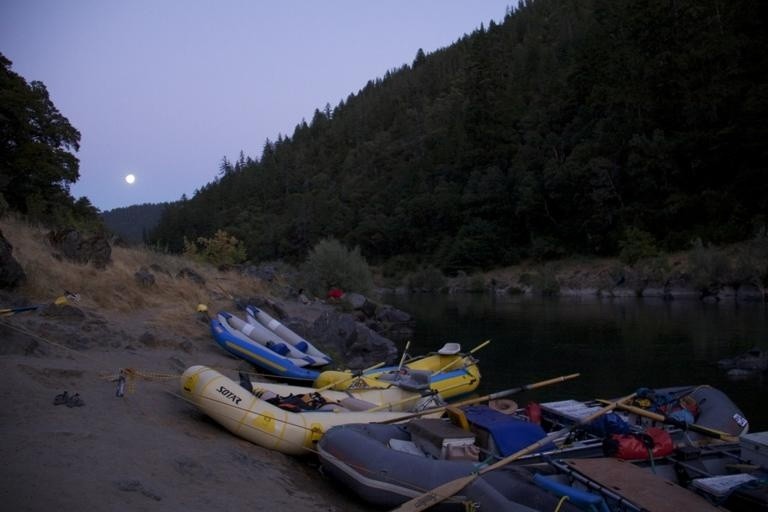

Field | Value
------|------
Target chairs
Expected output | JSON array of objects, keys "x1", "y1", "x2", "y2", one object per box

[
  {"x1": 400, "y1": 372, "x2": 430, "y2": 391},
  {"x1": 438, "y1": 342, "x2": 460, "y2": 355}
]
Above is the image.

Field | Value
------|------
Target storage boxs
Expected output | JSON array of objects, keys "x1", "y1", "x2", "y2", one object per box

[{"x1": 740, "y1": 432, "x2": 768, "y2": 470}]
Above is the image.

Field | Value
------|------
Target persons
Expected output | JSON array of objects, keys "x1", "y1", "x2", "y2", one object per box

[{"x1": 298, "y1": 289, "x2": 309, "y2": 305}]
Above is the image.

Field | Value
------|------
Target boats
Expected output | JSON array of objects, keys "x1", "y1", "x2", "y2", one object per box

[
  {"x1": 245, "y1": 303, "x2": 333, "y2": 366},
  {"x1": 312, "y1": 353, "x2": 480, "y2": 414},
  {"x1": 467, "y1": 431, "x2": 767, "y2": 512},
  {"x1": 217, "y1": 309, "x2": 315, "y2": 368},
  {"x1": 178, "y1": 365, "x2": 448, "y2": 457},
  {"x1": 315, "y1": 383, "x2": 749, "y2": 512},
  {"x1": 210, "y1": 319, "x2": 320, "y2": 380}
]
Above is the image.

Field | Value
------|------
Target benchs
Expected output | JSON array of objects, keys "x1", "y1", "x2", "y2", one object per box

[{"x1": 409, "y1": 419, "x2": 476, "y2": 461}]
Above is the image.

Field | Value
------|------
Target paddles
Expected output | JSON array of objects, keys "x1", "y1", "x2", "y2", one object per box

[{"x1": 381, "y1": 386, "x2": 651, "y2": 512}]
